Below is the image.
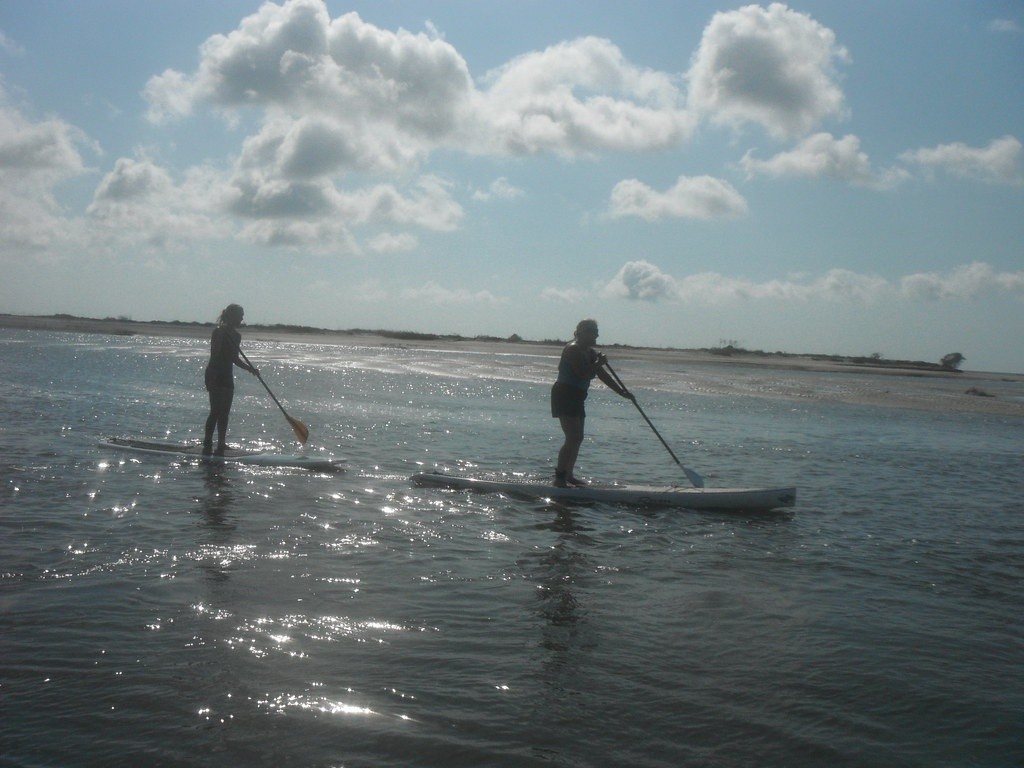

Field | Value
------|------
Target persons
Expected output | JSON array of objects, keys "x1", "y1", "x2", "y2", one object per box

[
  {"x1": 203, "y1": 304, "x2": 261, "y2": 455},
  {"x1": 551, "y1": 319, "x2": 635, "y2": 489}
]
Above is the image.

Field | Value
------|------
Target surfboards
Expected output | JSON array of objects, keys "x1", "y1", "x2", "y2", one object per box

[
  {"x1": 410, "y1": 471, "x2": 797, "y2": 514},
  {"x1": 98, "y1": 436, "x2": 349, "y2": 469}
]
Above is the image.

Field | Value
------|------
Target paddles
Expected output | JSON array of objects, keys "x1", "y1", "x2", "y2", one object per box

[
  {"x1": 227, "y1": 334, "x2": 309, "y2": 446},
  {"x1": 598, "y1": 352, "x2": 703, "y2": 488}
]
